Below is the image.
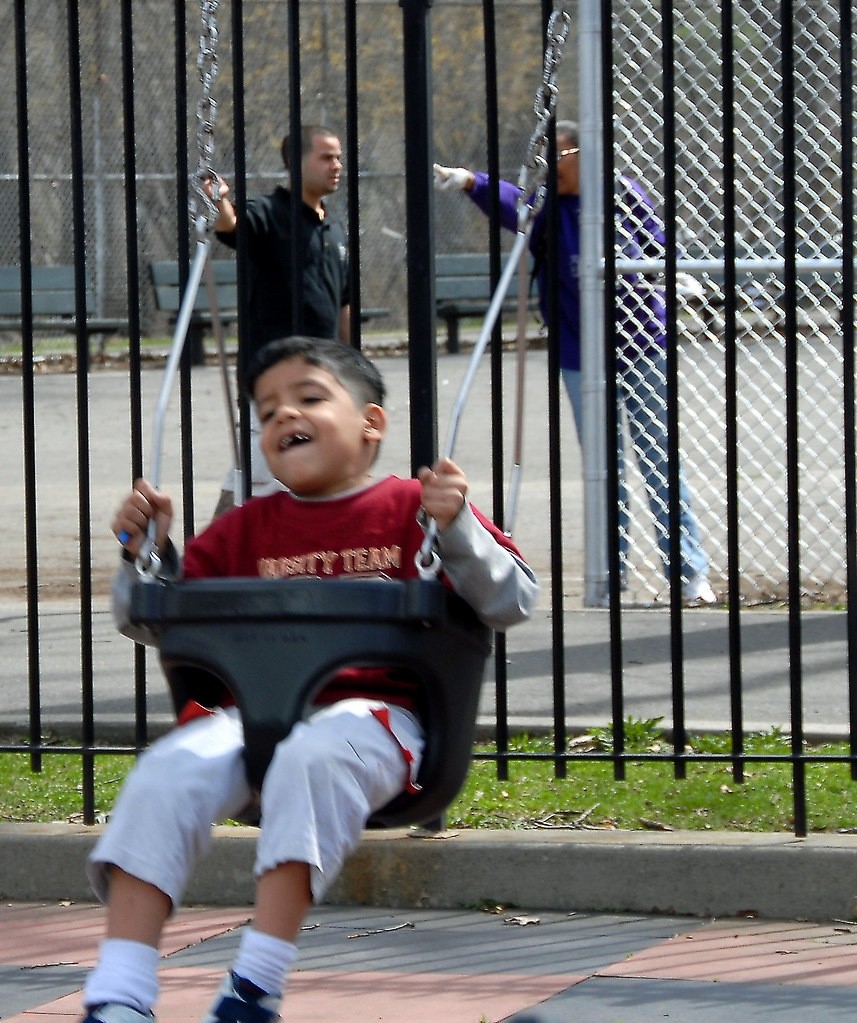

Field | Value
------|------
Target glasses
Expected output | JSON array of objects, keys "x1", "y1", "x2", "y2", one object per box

[{"x1": 557, "y1": 147, "x2": 580, "y2": 161}]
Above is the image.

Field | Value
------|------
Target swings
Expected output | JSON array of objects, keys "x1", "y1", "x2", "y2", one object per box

[{"x1": 126, "y1": 0, "x2": 575, "y2": 829}]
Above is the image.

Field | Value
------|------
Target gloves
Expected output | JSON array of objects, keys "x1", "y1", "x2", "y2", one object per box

[{"x1": 433, "y1": 164, "x2": 469, "y2": 192}]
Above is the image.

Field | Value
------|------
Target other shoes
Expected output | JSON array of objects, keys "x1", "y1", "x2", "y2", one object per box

[
  {"x1": 201, "y1": 970, "x2": 284, "y2": 1023},
  {"x1": 81, "y1": 1002, "x2": 155, "y2": 1023}
]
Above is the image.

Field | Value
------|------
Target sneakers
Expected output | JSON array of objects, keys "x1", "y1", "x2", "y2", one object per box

[{"x1": 680, "y1": 573, "x2": 717, "y2": 603}]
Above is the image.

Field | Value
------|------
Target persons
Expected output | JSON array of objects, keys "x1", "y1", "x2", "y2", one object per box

[
  {"x1": 204, "y1": 125, "x2": 349, "y2": 492},
  {"x1": 433, "y1": 119, "x2": 717, "y2": 606},
  {"x1": 79, "y1": 335, "x2": 538, "y2": 1023}
]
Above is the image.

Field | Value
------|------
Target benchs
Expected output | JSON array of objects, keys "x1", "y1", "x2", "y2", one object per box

[
  {"x1": 0, "y1": 263, "x2": 132, "y2": 361},
  {"x1": 432, "y1": 252, "x2": 537, "y2": 353},
  {"x1": 150, "y1": 257, "x2": 392, "y2": 367}
]
